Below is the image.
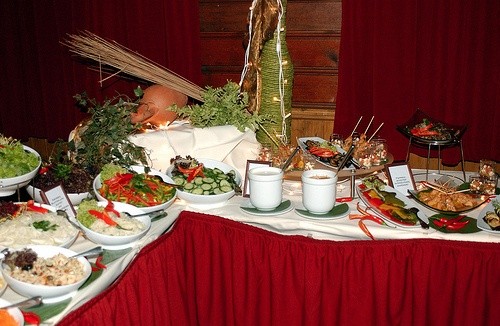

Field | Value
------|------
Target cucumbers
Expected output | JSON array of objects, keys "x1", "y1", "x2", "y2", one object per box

[{"x1": 171, "y1": 167, "x2": 233, "y2": 194}]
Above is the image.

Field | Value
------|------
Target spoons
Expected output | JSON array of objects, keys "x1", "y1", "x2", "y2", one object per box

[
  {"x1": 57, "y1": 210, "x2": 83, "y2": 231},
  {"x1": 410, "y1": 207, "x2": 430, "y2": 229}
]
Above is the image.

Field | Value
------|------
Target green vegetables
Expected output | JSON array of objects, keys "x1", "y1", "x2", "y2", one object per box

[
  {"x1": 56, "y1": 162, "x2": 72, "y2": 179},
  {"x1": 76, "y1": 197, "x2": 102, "y2": 228},
  {"x1": 33, "y1": 220, "x2": 60, "y2": 231},
  {"x1": 456, "y1": 180, "x2": 483, "y2": 195},
  {"x1": 0, "y1": 135, "x2": 41, "y2": 178},
  {"x1": 100, "y1": 163, "x2": 176, "y2": 207}
]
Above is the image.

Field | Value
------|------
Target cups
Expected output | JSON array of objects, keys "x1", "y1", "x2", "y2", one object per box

[
  {"x1": 370, "y1": 139, "x2": 388, "y2": 165},
  {"x1": 301, "y1": 169, "x2": 338, "y2": 214},
  {"x1": 354, "y1": 144, "x2": 373, "y2": 170},
  {"x1": 249, "y1": 167, "x2": 283, "y2": 211},
  {"x1": 330, "y1": 135, "x2": 343, "y2": 149}
]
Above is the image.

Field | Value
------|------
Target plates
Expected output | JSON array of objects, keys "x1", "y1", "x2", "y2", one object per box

[
  {"x1": 0, "y1": 252, "x2": 8, "y2": 298},
  {"x1": 407, "y1": 174, "x2": 489, "y2": 215},
  {"x1": 297, "y1": 136, "x2": 360, "y2": 170},
  {"x1": 477, "y1": 194, "x2": 500, "y2": 233},
  {"x1": 357, "y1": 179, "x2": 430, "y2": 228},
  {"x1": 396, "y1": 109, "x2": 468, "y2": 149},
  {"x1": 294, "y1": 199, "x2": 351, "y2": 219},
  {"x1": 240, "y1": 197, "x2": 294, "y2": 216}
]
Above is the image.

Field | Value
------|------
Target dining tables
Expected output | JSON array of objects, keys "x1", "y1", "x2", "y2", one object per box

[{"x1": 0, "y1": 155, "x2": 500, "y2": 326}]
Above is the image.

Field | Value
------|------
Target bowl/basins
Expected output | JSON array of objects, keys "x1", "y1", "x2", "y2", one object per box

[
  {"x1": 0, "y1": 202, "x2": 80, "y2": 249},
  {"x1": 26, "y1": 184, "x2": 96, "y2": 206},
  {"x1": 0, "y1": 298, "x2": 24, "y2": 326},
  {"x1": 77, "y1": 202, "x2": 151, "y2": 247},
  {"x1": 166, "y1": 158, "x2": 243, "y2": 204},
  {"x1": 93, "y1": 165, "x2": 177, "y2": 213},
  {"x1": 0, "y1": 144, "x2": 42, "y2": 197},
  {"x1": 0, "y1": 245, "x2": 92, "y2": 300}
]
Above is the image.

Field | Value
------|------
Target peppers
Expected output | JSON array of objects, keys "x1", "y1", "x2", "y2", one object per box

[
  {"x1": 22, "y1": 310, "x2": 53, "y2": 324},
  {"x1": 418, "y1": 123, "x2": 443, "y2": 135},
  {"x1": 425, "y1": 215, "x2": 468, "y2": 229},
  {"x1": 349, "y1": 203, "x2": 396, "y2": 239},
  {"x1": 28, "y1": 204, "x2": 54, "y2": 213},
  {"x1": 336, "y1": 197, "x2": 358, "y2": 202},
  {"x1": 88, "y1": 174, "x2": 159, "y2": 231},
  {"x1": 177, "y1": 163, "x2": 206, "y2": 182},
  {"x1": 92, "y1": 252, "x2": 106, "y2": 270}
]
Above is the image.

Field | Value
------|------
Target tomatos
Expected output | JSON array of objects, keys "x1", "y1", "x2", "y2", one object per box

[{"x1": 309, "y1": 147, "x2": 334, "y2": 157}]
